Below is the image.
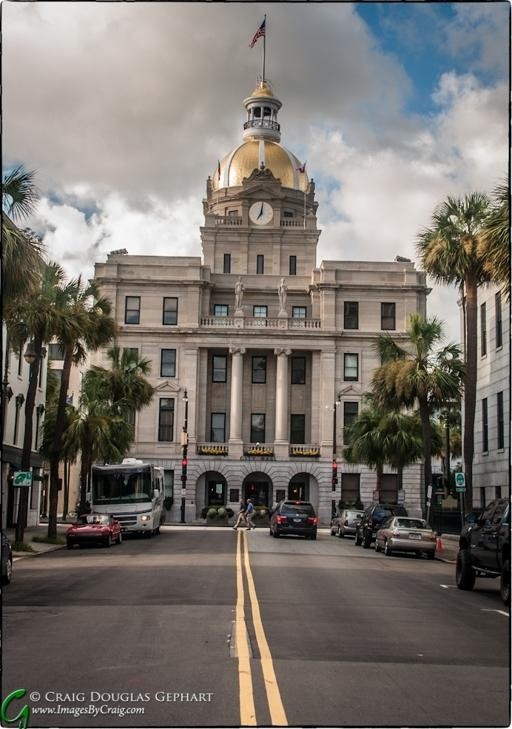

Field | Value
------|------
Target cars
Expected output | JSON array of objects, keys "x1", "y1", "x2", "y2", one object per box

[
  {"x1": 0, "y1": 530, "x2": 14, "y2": 584},
  {"x1": 64, "y1": 512, "x2": 124, "y2": 549},
  {"x1": 328, "y1": 507, "x2": 367, "y2": 539},
  {"x1": 373, "y1": 517, "x2": 439, "y2": 559}
]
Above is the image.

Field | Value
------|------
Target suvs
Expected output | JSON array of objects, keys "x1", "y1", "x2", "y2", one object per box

[
  {"x1": 454, "y1": 496, "x2": 511, "y2": 607},
  {"x1": 354, "y1": 503, "x2": 407, "y2": 549},
  {"x1": 268, "y1": 499, "x2": 318, "y2": 540}
]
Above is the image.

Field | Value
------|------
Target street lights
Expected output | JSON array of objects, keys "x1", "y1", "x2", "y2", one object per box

[
  {"x1": 437, "y1": 410, "x2": 452, "y2": 499},
  {"x1": 332, "y1": 392, "x2": 341, "y2": 530},
  {"x1": 178, "y1": 386, "x2": 190, "y2": 524},
  {"x1": 13, "y1": 325, "x2": 48, "y2": 543}
]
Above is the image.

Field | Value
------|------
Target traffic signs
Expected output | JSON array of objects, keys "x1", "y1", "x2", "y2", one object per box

[{"x1": 455, "y1": 487, "x2": 467, "y2": 493}]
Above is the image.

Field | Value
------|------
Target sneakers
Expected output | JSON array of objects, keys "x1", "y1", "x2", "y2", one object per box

[{"x1": 246, "y1": 525, "x2": 256, "y2": 530}]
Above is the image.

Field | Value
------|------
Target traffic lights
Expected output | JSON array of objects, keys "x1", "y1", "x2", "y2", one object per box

[
  {"x1": 182, "y1": 458, "x2": 188, "y2": 475},
  {"x1": 332, "y1": 461, "x2": 338, "y2": 477},
  {"x1": 333, "y1": 478, "x2": 338, "y2": 484},
  {"x1": 180, "y1": 475, "x2": 187, "y2": 481}
]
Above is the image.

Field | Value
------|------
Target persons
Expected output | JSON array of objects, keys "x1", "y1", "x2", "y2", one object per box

[
  {"x1": 233, "y1": 499, "x2": 248, "y2": 530},
  {"x1": 277, "y1": 278, "x2": 289, "y2": 311},
  {"x1": 244, "y1": 498, "x2": 256, "y2": 530},
  {"x1": 234, "y1": 276, "x2": 245, "y2": 308}
]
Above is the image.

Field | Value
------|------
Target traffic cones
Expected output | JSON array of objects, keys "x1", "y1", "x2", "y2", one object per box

[{"x1": 433, "y1": 540, "x2": 445, "y2": 553}]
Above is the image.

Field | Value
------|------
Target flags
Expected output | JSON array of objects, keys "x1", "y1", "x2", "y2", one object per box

[{"x1": 249, "y1": 19, "x2": 264, "y2": 48}]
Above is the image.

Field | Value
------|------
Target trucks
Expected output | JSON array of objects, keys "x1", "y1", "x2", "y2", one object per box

[{"x1": 90, "y1": 455, "x2": 168, "y2": 539}]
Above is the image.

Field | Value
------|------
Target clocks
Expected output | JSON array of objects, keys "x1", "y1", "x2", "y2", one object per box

[{"x1": 249, "y1": 200, "x2": 274, "y2": 227}]
